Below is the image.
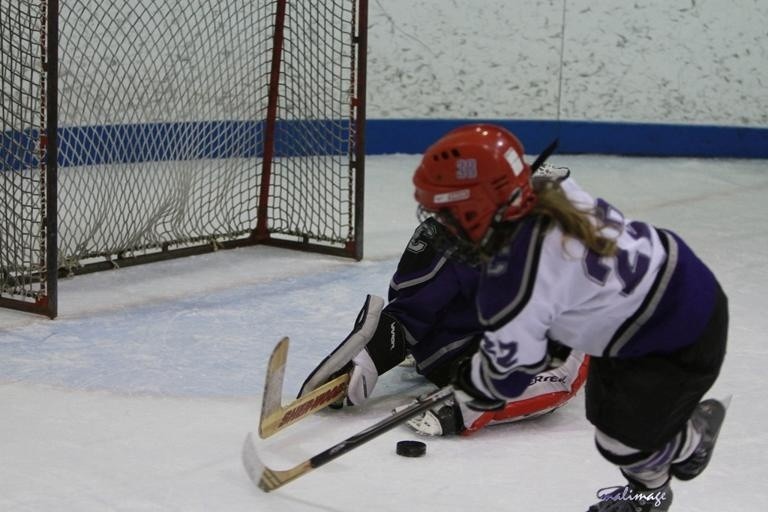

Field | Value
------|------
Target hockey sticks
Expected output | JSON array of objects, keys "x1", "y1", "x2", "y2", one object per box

[
  {"x1": 259, "y1": 338, "x2": 351, "y2": 440},
  {"x1": 243, "y1": 386, "x2": 454, "y2": 493}
]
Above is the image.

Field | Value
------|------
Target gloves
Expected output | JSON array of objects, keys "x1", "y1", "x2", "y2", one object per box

[
  {"x1": 324, "y1": 309, "x2": 407, "y2": 411},
  {"x1": 453, "y1": 351, "x2": 512, "y2": 438}
]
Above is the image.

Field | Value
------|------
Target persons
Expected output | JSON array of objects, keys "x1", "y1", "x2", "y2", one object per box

[
  {"x1": 295, "y1": 216, "x2": 591, "y2": 438},
  {"x1": 413, "y1": 124, "x2": 728, "y2": 512}
]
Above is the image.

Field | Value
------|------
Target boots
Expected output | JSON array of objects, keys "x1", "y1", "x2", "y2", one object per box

[
  {"x1": 588, "y1": 465, "x2": 674, "y2": 511},
  {"x1": 673, "y1": 398, "x2": 723, "y2": 482}
]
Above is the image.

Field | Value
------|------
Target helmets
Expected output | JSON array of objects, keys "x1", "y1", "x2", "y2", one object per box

[{"x1": 412, "y1": 123, "x2": 538, "y2": 267}]
